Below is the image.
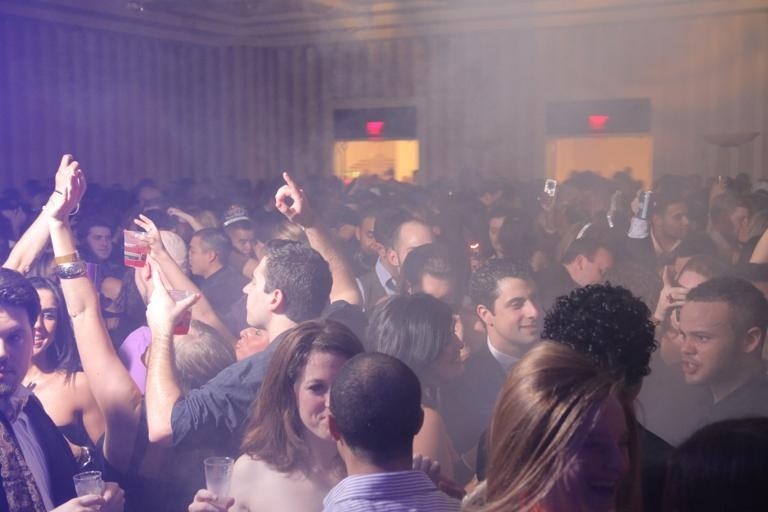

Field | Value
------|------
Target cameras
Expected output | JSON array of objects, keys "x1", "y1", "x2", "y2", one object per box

[{"x1": 544, "y1": 178, "x2": 557, "y2": 197}]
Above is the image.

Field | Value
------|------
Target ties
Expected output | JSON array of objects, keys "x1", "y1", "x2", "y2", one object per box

[{"x1": 387, "y1": 279, "x2": 402, "y2": 293}]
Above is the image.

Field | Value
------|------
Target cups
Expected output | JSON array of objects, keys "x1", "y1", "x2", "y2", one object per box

[
  {"x1": 72, "y1": 470, "x2": 104, "y2": 511},
  {"x1": 716, "y1": 175, "x2": 728, "y2": 190},
  {"x1": 203, "y1": 457, "x2": 234, "y2": 504},
  {"x1": 123, "y1": 230, "x2": 149, "y2": 267},
  {"x1": 168, "y1": 289, "x2": 194, "y2": 335}
]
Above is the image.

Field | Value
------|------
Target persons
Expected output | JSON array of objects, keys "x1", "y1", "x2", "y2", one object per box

[{"x1": 0, "y1": 153, "x2": 768, "y2": 512}]
